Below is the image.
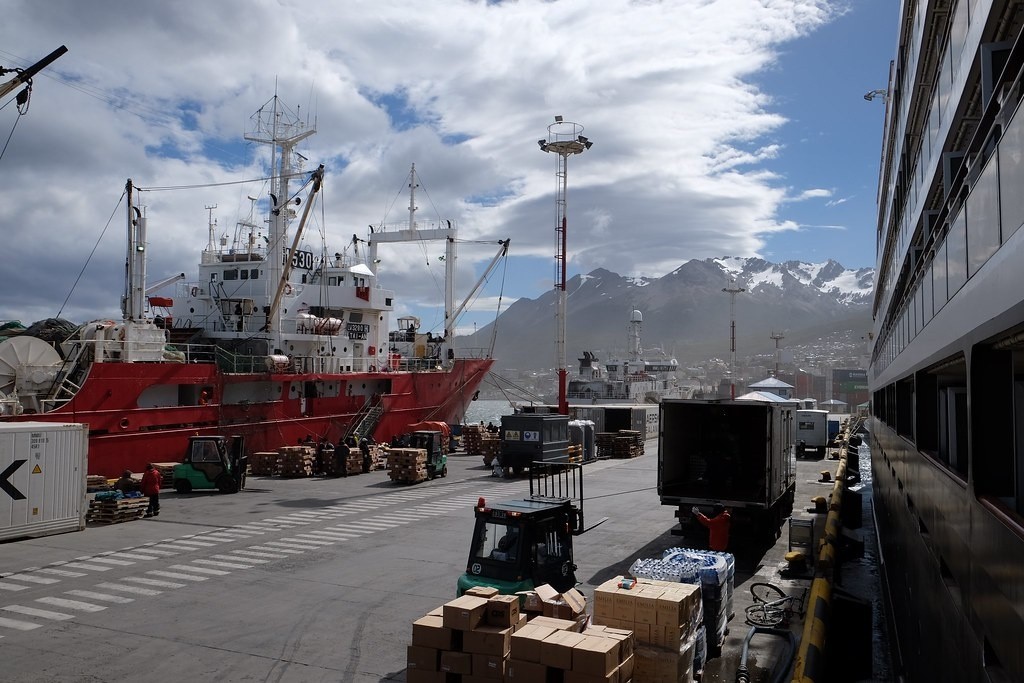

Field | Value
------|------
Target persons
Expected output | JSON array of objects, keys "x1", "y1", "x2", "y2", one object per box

[
  {"x1": 293, "y1": 432, "x2": 411, "y2": 478},
  {"x1": 480, "y1": 419, "x2": 498, "y2": 433},
  {"x1": 690, "y1": 503, "x2": 730, "y2": 551},
  {"x1": 198, "y1": 390, "x2": 207, "y2": 406},
  {"x1": 591, "y1": 393, "x2": 598, "y2": 404},
  {"x1": 141, "y1": 462, "x2": 162, "y2": 518},
  {"x1": 408, "y1": 324, "x2": 415, "y2": 339},
  {"x1": 234, "y1": 299, "x2": 253, "y2": 331},
  {"x1": 115, "y1": 468, "x2": 138, "y2": 497},
  {"x1": 139, "y1": 493, "x2": 140, "y2": 495}
]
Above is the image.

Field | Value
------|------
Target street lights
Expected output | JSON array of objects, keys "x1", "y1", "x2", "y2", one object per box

[
  {"x1": 722, "y1": 288, "x2": 745, "y2": 400},
  {"x1": 538, "y1": 134, "x2": 593, "y2": 415},
  {"x1": 770, "y1": 333, "x2": 784, "y2": 380}
]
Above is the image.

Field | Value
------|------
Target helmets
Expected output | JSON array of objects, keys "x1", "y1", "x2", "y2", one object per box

[
  {"x1": 713, "y1": 503, "x2": 724, "y2": 508},
  {"x1": 362, "y1": 438, "x2": 368, "y2": 442}
]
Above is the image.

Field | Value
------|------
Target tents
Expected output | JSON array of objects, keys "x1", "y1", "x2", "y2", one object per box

[{"x1": 856, "y1": 401, "x2": 869, "y2": 416}]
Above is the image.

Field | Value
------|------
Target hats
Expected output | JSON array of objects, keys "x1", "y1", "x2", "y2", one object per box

[
  {"x1": 202, "y1": 390, "x2": 207, "y2": 398},
  {"x1": 123, "y1": 470, "x2": 133, "y2": 476}
]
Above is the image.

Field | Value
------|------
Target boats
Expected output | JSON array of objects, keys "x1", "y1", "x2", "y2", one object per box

[
  {"x1": 0, "y1": 74, "x2": 510, "y2": 480},
  {"x1": 514, "y1": 307, "x2": 684, "y2": 440}
]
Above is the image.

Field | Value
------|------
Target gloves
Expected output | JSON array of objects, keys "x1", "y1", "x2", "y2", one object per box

[{"x1": 692, "y1": 506, "x2": 699, "y2": 515}]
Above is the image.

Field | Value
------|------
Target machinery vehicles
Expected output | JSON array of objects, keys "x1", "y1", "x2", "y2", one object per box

[
  {"x1": 172, "y1": 435, "x2": 248, "y2": 494},
  {"x1": 456, "y1": 461, "x2": 609, "y2": 615},
  {"x1": 411, "y1": 431, "x2": 447, "y2": 480}
]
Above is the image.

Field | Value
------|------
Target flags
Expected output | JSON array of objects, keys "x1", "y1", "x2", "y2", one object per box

[{"x1": 147, "y1": 295, "x2": 173, "y2": 309}]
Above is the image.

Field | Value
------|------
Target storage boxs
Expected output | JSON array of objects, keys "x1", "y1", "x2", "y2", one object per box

[
  {"x1": 252, "y1": 443, "x2": 427, "y2": 483},
  {"x1": 462, "y1": 424, "x2": 500, "y2": 465},
  {"x1": 402, "y1": 574, "x2": 700, "y2": 682}
]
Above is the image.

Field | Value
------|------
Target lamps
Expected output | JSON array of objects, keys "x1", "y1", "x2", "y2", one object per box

[{"x1": 863, "y1": 88, "x2": 887, "y2": 102}]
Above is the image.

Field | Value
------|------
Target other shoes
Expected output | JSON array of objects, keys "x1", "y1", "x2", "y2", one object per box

[
  {"x1": 153, "y1": 512, "x2": 159, "y2": 516},
  {"x1": 145, "y1": 514, "x2": 152, "y2": 518}
]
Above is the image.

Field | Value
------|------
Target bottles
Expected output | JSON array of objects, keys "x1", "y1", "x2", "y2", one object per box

[{"x1": 632, "y1": 547, "x2": 734, "y2": 669}]
